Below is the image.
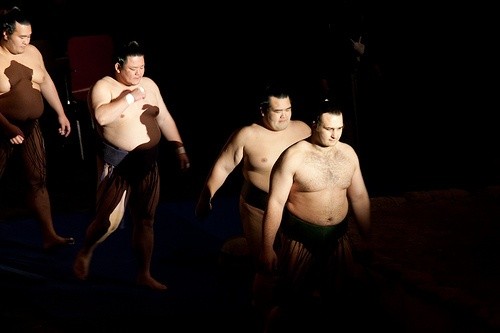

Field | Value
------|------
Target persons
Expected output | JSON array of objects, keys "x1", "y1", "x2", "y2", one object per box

[
  {"x1": 0, "y1": 7, "x2": 77, "y2": 252},
  {"x1": 194, "y1": 88, "x2": 312, "y2": 306},
  {"x1": 257, "y1": 103, "x2": 374, "y2": 333},
  {"x1": 72, "y1": 41, "x2": 191, "y2": 292}
]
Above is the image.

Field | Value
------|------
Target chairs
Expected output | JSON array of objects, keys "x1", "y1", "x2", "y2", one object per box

[{"x1": 65, "y1": 36, "x2": 113, "y2": 161}]
